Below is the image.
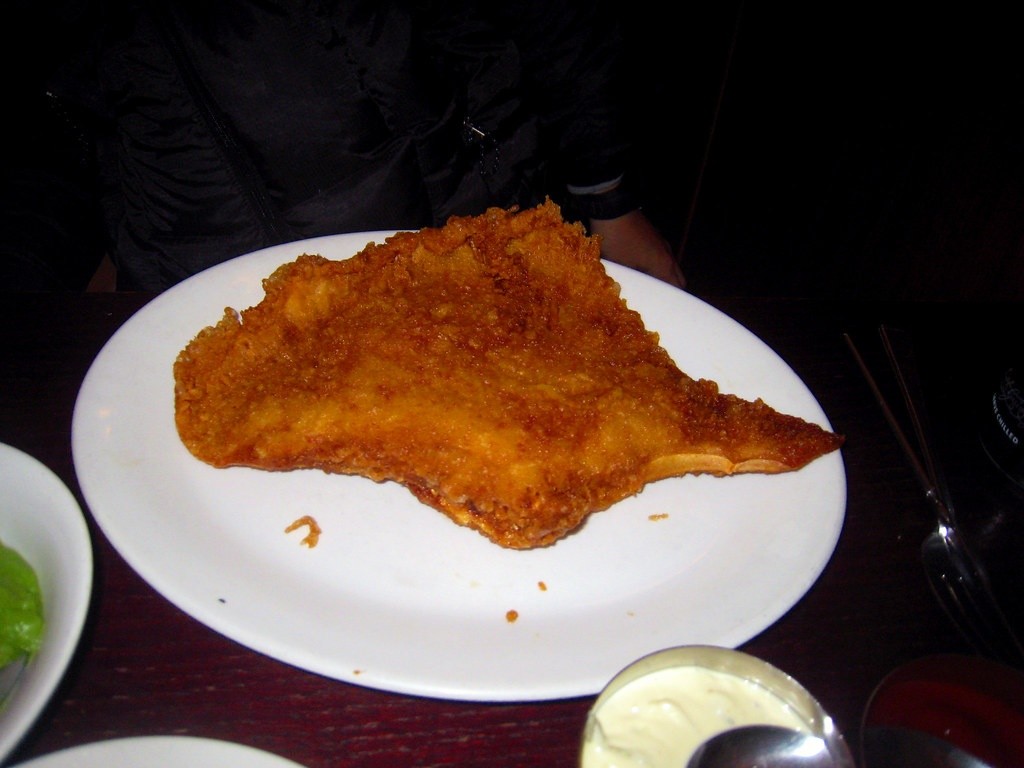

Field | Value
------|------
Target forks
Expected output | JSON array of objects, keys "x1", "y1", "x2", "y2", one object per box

[{"x1": 840, "y1": 317, "x2": 1024, "y2": 668}]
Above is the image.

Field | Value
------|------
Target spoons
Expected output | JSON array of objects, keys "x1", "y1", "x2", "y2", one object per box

[{"x1": 686, "y1": 725, "x2": 832, "y2": 767}]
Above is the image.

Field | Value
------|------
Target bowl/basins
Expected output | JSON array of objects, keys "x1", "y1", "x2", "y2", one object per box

[
  {"x1": 0, "y1": 441, "x2": 95, "y2": 763},
  {"x1": 859, "y1": 654, "x2": 1024, "y2": 768}
]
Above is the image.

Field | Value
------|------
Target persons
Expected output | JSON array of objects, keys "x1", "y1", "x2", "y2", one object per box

[{"x1": 98, "y1": 0, "x2": 690, "y2": 292}]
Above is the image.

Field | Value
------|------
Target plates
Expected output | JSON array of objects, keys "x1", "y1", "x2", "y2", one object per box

[
  {"x1": 7, "y1": 734, "x2": 308, "y2": 768},
  {"x1": 70, "y1": 227, "x2": 850, "y2": 706}
]
